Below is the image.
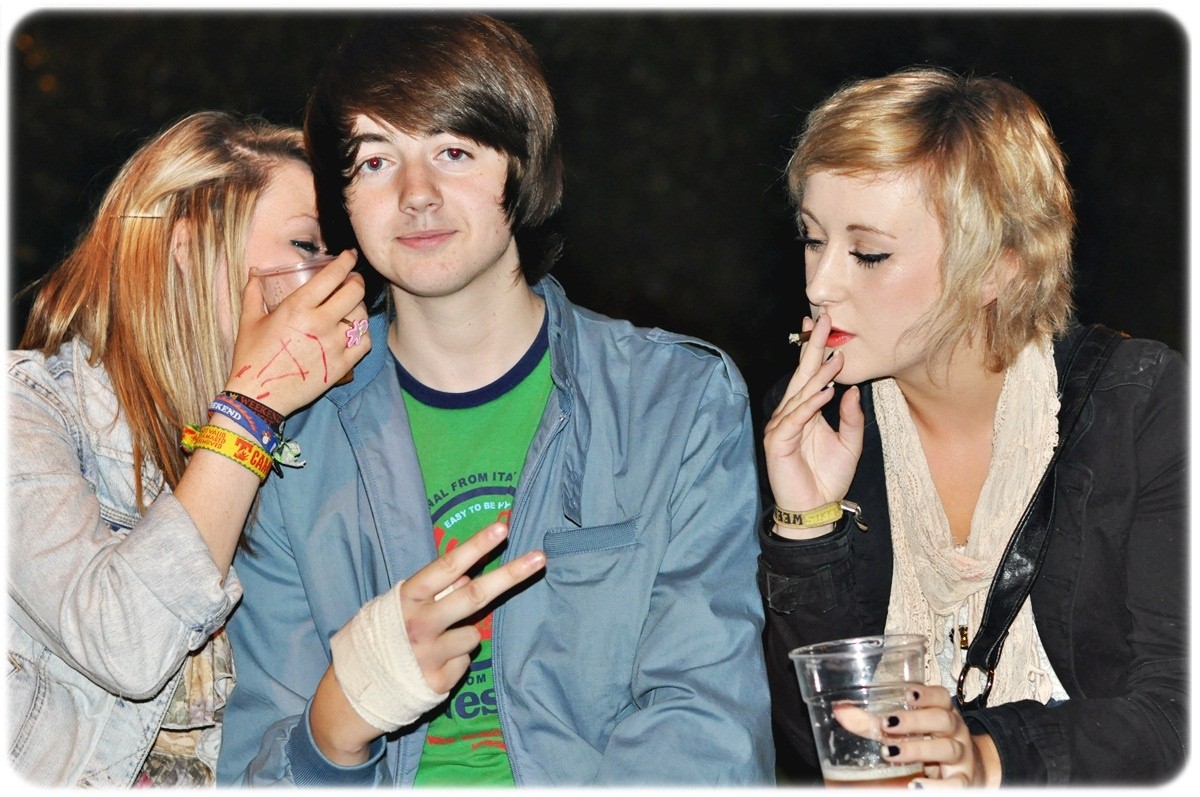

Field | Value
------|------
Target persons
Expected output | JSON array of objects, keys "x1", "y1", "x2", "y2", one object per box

[
  {"x1": 9, "y1": 114, "x2": 372, "y2": 789},
  {"x1": 215, "y1": 12, "x2": 773, "y2": 787},
  {"x1": 755, "y1": 69, "x2": 1191, "y2": 791}
]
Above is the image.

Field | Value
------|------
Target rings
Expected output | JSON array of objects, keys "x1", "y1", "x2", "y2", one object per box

[{"x1": 341, "y1": 318, "x2": 369, "y2": 348}]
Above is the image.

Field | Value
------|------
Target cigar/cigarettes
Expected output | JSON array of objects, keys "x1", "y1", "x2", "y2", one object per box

[{"x1": 789, "y1": 331, "x2": 811, "y2": 344}]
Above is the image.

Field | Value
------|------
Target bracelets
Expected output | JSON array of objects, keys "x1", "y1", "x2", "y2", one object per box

[
  {"x1": 773, "y1": 501, "x2": 867, "y2": 532},
  {"x1": 181, "y1": 425, "x2": 272, "y2": 485},
  {"x1": 209, "y1": 391, "x2": 305, "y2": 479}
]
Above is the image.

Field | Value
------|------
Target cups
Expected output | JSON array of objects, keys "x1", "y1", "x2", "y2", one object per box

[
  {"x1": 789, "y1": 633, "x2": 929, "y2": 788},
  {"x1": 252, "y1": 256, "x2": 354, "y2": 386}
]
